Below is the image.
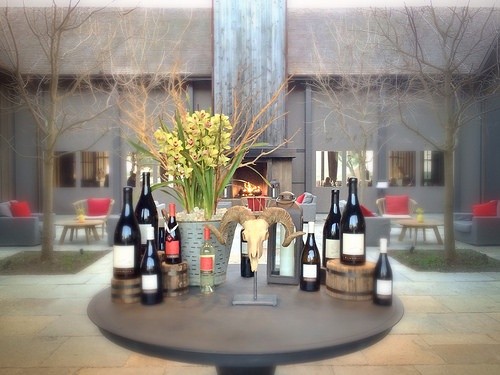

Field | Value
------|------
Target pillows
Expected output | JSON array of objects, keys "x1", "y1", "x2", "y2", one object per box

[
  {"x1": 247, "y1": 197, "x2": 265, "y2": 211},
  {"x1": 386, "y1": 195, "x2": 409, "y2": 214},
  {"x1": 10, "y1": 201, "x2": 31, "y2": 217},
  {"x1": 296, "y1": 194, "x2": 305, "y2": 203},
  {"x1": 472, "y1": 200, "x2": 497, "y2": 216},
  {"x1": 360, "y1": 205, "x2": 374, "y2": 216},
  {"x1": 86, "y1": 198, "x2": 110, "y2": 215}
]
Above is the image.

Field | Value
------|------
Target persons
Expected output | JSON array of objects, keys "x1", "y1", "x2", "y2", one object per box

[{"x1": 324, "y1": 177, "x2": 331, "y2": 187}]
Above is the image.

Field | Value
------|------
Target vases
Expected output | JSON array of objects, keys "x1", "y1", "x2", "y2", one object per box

[{"x1": 172, "y1": 208, "x2": 238, "y2": 286}]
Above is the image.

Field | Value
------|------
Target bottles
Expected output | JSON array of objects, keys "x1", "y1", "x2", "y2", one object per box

[
  {"x1": 322, "y1": 189, "x2": 342, "y2": 268},
  {"x1": 112, "y1": 186, "x2": 140, "y2": 280},
  {"x1": 134, "y1": 172, "x2": 160, "y2": 254},
  {"x1": 372, "y1": 238, "x2": 393, "y2": 307},
  {"x1": 240, "y1": 228, "x2": 254, "y2": 278},
  {"x1": 138, "y1": 226, "x2": 162, "y2": 306},
  {"x1": 163, "y1": 203, "x2": 182, "y2": 265},
  {"x1": 300, "y1": 221, "x2": 321, "y2": 292},
  {"x1": 339, "y1": 177, "x2": 367, "y2": 265},
  {"x1": 200, "y1": 229, "x2": 215, "y2": 295}
]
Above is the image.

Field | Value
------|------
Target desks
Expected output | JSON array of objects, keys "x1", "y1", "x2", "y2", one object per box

[
  {"x1": 398, "y1": 220, "x2": 445, "y2": 246},
  {"x1": 86, "y1": 264, "x2": 404, "y2": 375},
  {"x1": 58, "y1": 220, "x2": 105, "y2": 244}
]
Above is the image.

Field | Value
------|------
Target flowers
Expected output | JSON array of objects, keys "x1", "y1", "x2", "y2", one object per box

[{"x1": 127, "y1": 103, "x2": 269, "y2": 220}]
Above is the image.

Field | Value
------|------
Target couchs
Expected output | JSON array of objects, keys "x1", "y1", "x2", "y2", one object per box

[
  {"x1": 105, "y1": 201, "x2": 166, "y2": 246},
  {"x1": 453, "y1": 200, "x2": 500, "y2": 245},
  {"x1": 338, "y1": 200, "x2": 390, "y2": 247},
  {"x1": 297, "y1": 192, "x2": 317, "y2": 223},
  {"x1": 0, "y1": 201, "x2": 56, "y2": 246}
]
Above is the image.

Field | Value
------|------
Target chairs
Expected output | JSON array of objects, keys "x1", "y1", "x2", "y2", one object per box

[
  {"x1": 241, "y1": 197, "x2": 271, "y2": 208},
  {"x1": 375, "y1": 198, "x2": 418, "y2": 238},
  {"x1": 72, "y1": 198, "x2": 115, "y2": 219}
]
